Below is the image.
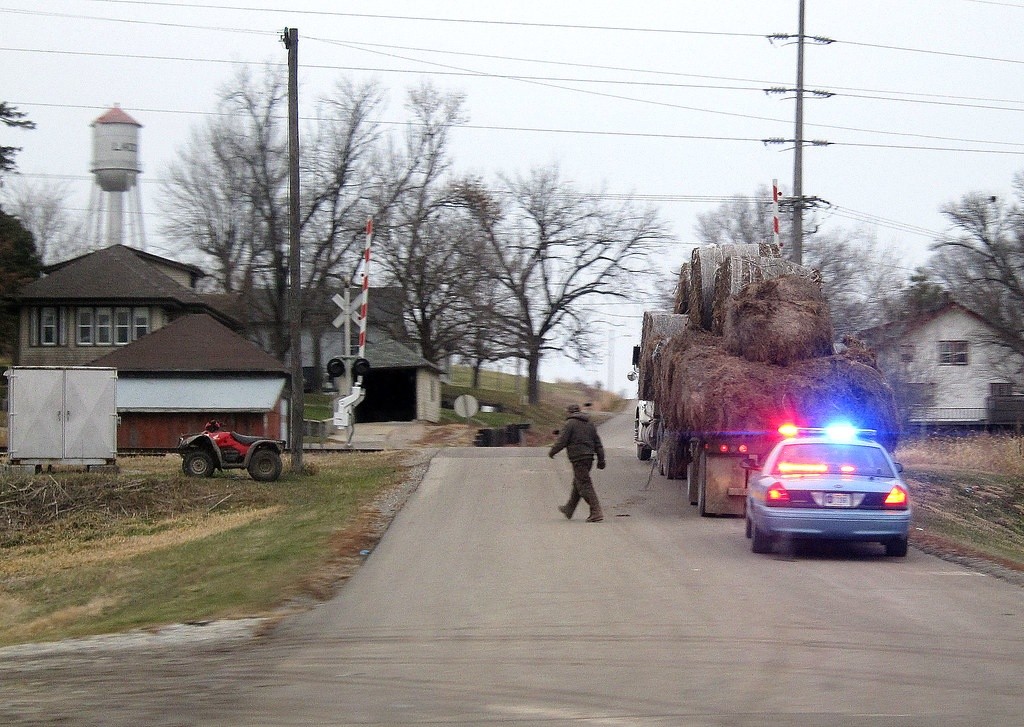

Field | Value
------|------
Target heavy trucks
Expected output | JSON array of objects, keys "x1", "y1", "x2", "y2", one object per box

[{"x1": 631, "y1": 249, "x2": 798, "y2": 519}]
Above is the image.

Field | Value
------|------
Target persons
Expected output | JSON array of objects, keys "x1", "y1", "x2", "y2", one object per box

[{"x1": 548, "y1": 404, "x2": 606, "y2": 521}]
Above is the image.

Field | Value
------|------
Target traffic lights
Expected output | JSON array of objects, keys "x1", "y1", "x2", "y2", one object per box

[{"x1": 325, "y1": 358, "x2": 371, "y2": 377}]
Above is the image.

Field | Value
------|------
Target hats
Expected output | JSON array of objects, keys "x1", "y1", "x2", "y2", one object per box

[{"x1": 567, "y1": 404, "x2": 580, "y2": 412}]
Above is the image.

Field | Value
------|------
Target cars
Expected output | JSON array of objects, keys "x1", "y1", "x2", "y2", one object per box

[{"x1": 743, "y1": 420, "x2": 914, "y2": 560}]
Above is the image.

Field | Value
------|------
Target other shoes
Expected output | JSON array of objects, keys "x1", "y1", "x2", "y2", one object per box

[
  {"x1": 558, "y1": 504, "x2": 576, "y2": 519},
  {"x1": 586, "y1": 508, "x2": 605, "y2": 522}
]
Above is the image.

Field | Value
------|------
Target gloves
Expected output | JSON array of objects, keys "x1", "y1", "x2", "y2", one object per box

[
  {"x1": 548, "y1": 446, "x2": 562, "y2": 459},
  {"x1": 595, "y1": 460, "x2": 606, "y2": 469}
]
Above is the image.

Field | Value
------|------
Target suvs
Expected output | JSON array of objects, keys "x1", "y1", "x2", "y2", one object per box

[{"x1": 174, "y1": 421, "x2": 287, "y2": 483}]
